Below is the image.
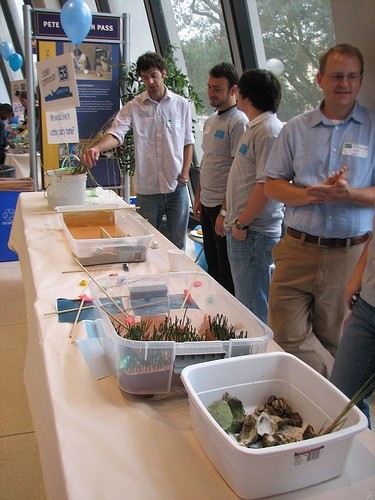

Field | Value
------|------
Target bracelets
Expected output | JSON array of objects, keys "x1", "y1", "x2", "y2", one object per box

[{"x1": 176, "y1": 173, "x2": 189, "y2": 186}]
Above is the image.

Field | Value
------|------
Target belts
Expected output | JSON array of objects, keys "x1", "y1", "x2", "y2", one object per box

[{"x1": 287, "y1": 226, "x2": 372, "y2": 248}]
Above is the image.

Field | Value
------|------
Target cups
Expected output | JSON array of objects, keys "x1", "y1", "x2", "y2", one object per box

[{"x1": 168, "y1": 249, "x2": 184, "y2": 272}]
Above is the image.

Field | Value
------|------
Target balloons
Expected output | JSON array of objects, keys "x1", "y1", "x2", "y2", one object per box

[
  {"x1": 0, "y1": 41, "x2": 23, "y2": 73},
  {"x1": 60, "y1": 0, "x2": 93, "y2": 48}
]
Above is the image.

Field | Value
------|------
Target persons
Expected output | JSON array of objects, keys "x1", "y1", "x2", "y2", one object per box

[
  {"x1": 192, "y1": 62, "x2": 250, "y2": 297},
  {"x1": 224, "y1": 68, "x2": 284, "y2": 326},
  {"x1": 81, "y1": 51, "x2": 196, "y2": 255},
  {"x1": 73, "y1": 49, "x2": 110, "y2": 72},
  {"x1": 263, "y1": 44, "x2": 375, "y2": 382},
  {"x1": 328, "y1": 213, "x2": 375, "y2": 430},
  {"x1": 0, "y1": 90, "x2": 40, "y2": 170}
]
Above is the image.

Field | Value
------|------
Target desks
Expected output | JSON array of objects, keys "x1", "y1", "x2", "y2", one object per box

[
  {"x1": 0, "y1": 191, "x2": 32, "y2": 262},
  {"x1": 7, "y1": 190, "x2": 375, "y2": 500},
  {"x1": 3, "y1": 142, "x2": 42, "y2": 190}
]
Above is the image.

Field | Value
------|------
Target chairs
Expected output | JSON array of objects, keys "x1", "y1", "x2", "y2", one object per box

[{"x1": 188, "y1": 225, "x2": 206, "y2": 264}]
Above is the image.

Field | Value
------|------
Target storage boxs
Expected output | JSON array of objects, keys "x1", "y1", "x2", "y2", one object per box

[
  {"x1": 88, "y1": 270, "x2": 273, "y2": 395},
  {"x1": 181, "y1": 351, "x2": 369, "y2": 500},
  {"x1": 60, "y1": 208, "x2": 153, "y2": 266},
  {"x1": 0, "y1": 177, "x2": 34, "y2": 191}
]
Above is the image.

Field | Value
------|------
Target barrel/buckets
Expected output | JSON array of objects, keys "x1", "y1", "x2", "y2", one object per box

[{"x1": 44, "y1": 167, "x2": 86, "y2": 211}]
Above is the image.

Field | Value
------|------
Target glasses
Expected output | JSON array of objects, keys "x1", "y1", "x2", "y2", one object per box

[{"x1": 323, "y1": 72, "x2": 362, "y2": 81}]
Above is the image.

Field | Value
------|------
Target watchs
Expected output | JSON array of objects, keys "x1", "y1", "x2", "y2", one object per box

[
  {"x1": 235, "y1": 218, "x2": 249, "y2": 231},
  {"x1": 219, "y1": 208, "x2": 227, "y2": 217}
]
